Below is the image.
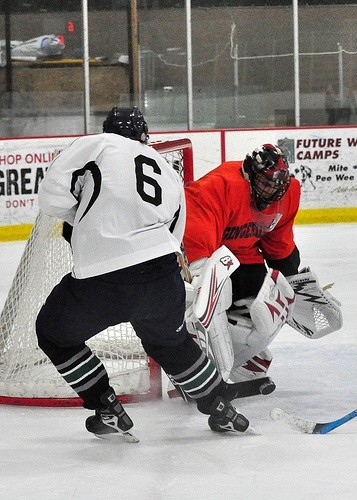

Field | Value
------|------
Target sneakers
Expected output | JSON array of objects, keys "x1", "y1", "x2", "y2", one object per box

[
  {"x1": 196, "y1": 397, "x2": 260, "y2": 436},
  {"x1": 81, "y1": 386, "x2": 143, "y2": 443}
]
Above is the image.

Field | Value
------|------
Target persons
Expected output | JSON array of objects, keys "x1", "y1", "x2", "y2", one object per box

[
  {"x1": 175, "y1": 142, "x2": 301, "y2": 397},
  {"x1": 34, "y1": 105, "x2": 250, "y2": 439}
]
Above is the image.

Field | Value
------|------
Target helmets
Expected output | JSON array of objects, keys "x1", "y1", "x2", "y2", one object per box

[
  {"x1": 241, "y1": 143, "x2": 291, "y2": 212},
  {"x1": 101, "y1": 106, "x2": 149, "y2": 146}
]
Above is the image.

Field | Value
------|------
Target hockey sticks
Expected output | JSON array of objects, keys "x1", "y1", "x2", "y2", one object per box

[{"x1": 269, "y1": 406, "x2": 357, "y2": 435}]
